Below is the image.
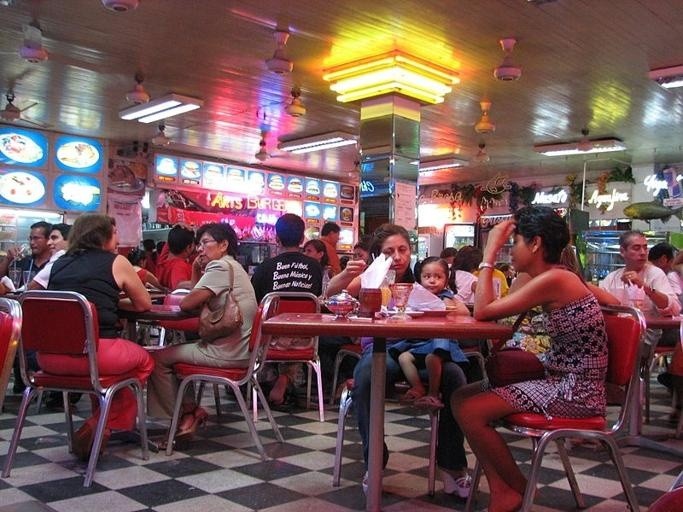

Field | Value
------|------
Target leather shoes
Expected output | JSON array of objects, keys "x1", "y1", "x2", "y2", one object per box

[{"x1": 488, "y1": 491, "x2": 523, "y2": 511}]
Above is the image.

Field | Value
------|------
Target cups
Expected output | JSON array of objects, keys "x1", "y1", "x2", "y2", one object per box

[
  {"x1": 358, "y1": 287, "x2": 381, "y2": 318},
  {"x1": 8, "y1": 265, "x2": 21, "y2": 289},
  {"x1": 22, "y1": 271, "x2": 34, "y2": 286}
]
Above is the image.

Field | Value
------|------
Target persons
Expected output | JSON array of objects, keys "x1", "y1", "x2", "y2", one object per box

[
  {"x1": 0, "y1": 213, "x2": 682, "y2": 439},
  {"x1": 322, "y1": 223, "x2": 481, "y2": 499},
  {"x1": 34, "y1": 212, "x2": 153, "y2": 462},
  {"x1": 449, "y1": 201, "x2": 607, "y2": 512}
]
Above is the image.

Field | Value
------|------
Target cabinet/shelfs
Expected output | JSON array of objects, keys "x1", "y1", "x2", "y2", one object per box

[{"x1": 582, "y1": 227, "x2": 682, "y2": 282}]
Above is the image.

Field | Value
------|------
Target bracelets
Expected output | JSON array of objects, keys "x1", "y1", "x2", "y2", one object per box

[{"x1": 477, "y1": 262, "x2": 494, "y2": 271}]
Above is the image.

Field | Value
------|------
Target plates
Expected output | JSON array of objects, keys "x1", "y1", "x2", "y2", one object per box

[
  {"x1": 0, "y1": 127, "x2": 102, "y2": 215},
  {"x1": 387, "y1": 311, "x2": 425, "y2": 318},
  {"x1": 416, "y1": 309, "x2": 457, "y2": 317}
]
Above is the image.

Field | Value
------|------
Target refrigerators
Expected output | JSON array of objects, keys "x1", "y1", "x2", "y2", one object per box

[{"x1": 442, "y1": 222, "x2": 476, "y2": 254}]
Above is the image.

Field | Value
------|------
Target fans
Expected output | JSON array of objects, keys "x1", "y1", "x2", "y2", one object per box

[
  {"x1": 0, "y1": 90, "x2": 52, "y2": 130},
  {"x1": 100, "y1": 0, "x2": 142, "y2": 16},
  {"x1": 282, "y1": 88, "x2": 310, "y2": 120},
  {"x1": 571, "y1": 125, "x2": 599, "y2": 153},
  {"x1": 490, "y1": 34, "x2": 525, "y2": 83},
  {"x1": 470, "y1": 140, "x2": 499, "y2": 166},
  {"x1": 123, "y1": 67, "x2": 149, "y2": 107},
  {"x1": 473, "y1": 95, "x2": 495, "y2": 136},
  {"x1": 0, "y1": 18, "x2": 103, "y2": 74},
  {"x1": 261, "y1": 26, "x2": 297, "y2": 78},
  {"x1": 251, "y1": 131, "x2": 270, "y2": 168}
]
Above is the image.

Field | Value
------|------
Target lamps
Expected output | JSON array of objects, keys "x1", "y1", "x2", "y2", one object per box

[
  {"x1": 645, "y1": 63, "x2": 683, "y2": 94},
  {"x1": 116, "y1": 92, "x2": 205, "y2": 125},
  {"x1": 320, "y1": 47, "x2": 462, "y2": 107},
  {"x1": 533, "y1": 136, "x2": 624, "y2": 160},
  {"x1": 412, "y1": 152, "x2": 472, "y2": 176},
  {"x1": 274, "y1": 128, "x2": 358, "y2": 155}
]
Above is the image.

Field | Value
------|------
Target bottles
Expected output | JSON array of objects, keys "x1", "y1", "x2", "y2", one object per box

[
  {"x1": 591, "y1": 267, "x2": 599, "y2": 286},
  {"x1": 322, "y1": 291, "x2": 362, "y2": 321}
]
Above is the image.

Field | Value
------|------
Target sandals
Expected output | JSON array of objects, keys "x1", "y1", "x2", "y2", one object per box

[
  {"x1": 440, "y1": 466, "x2": 475, "y2": 497},
  {"x1": 415, "y1": 396, "x2": 445, "y2": 409},
  {"x1": 400, "y1": 386, "x2": 422, "y2": 405},
  {"x1": 362, "y1": 468, "x2": 384, "y2": 501},
  {"x1": 175, "y1": 404, "x2": 210, "y2": 442}
]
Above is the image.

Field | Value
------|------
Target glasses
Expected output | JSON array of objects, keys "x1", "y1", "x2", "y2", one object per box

[{"x1": 196, "y1": 239, "x2": 217, "y2": 251}]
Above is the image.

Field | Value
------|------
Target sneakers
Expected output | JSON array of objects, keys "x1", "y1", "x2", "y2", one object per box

[{"x1": 71, "y1": 422, "x2": 92, "y2": 463}]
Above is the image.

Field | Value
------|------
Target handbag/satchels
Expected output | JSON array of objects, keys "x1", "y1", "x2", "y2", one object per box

[
  {"x1": 485, "y1": 346, "x2": 545, "y2": 383},
  {"x1": 199, "y1": 293, "x2": 244, "y2": 340}
]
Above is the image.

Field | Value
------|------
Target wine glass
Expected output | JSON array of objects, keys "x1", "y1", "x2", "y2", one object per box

[{"x1": 388, "y1": 282, "x2": 413, "y2": 321}]
